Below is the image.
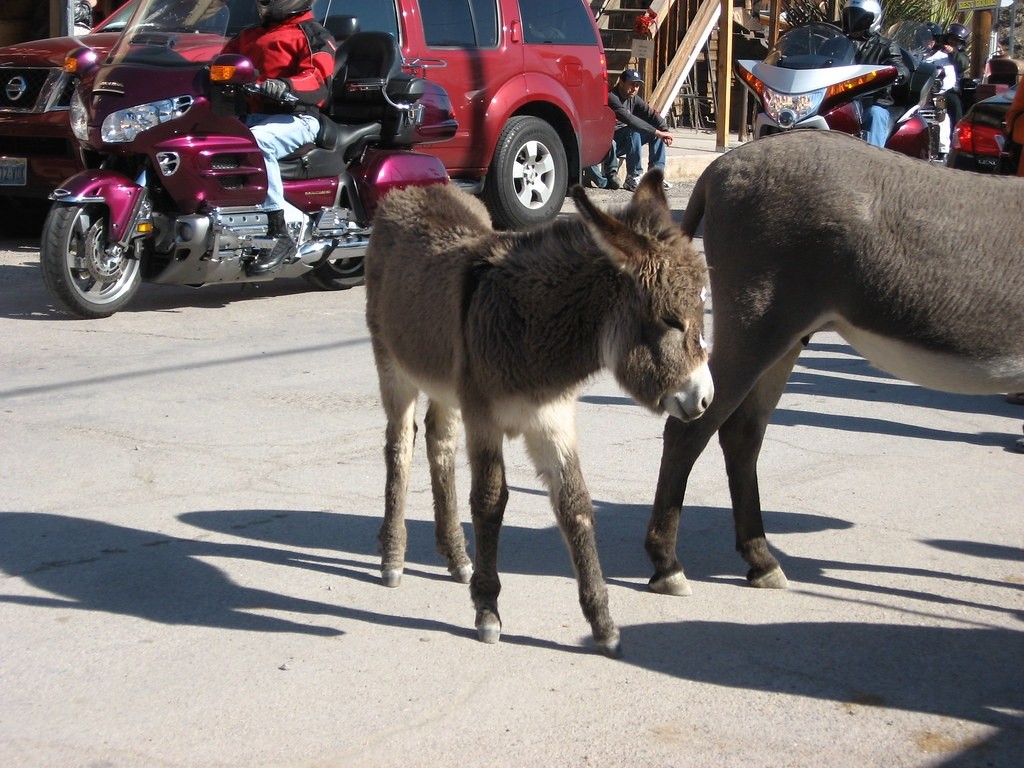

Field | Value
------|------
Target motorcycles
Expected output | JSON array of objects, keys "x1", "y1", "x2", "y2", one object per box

[
  {"x1": 37, "y1": 1, "x2": 459, "y2": 321},
  {"x1": 733, "y1": 22, "x2": 939, "y2": 161}
]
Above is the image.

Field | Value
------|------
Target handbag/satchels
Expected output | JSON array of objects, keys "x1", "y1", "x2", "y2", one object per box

[{"x1": 991, "y1": 111, "x2": 1023, "y2": 175}]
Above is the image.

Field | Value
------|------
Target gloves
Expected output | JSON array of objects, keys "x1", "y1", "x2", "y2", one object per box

[{"x1": 261, "y1": 78, "x2": 290, "y2": 101}]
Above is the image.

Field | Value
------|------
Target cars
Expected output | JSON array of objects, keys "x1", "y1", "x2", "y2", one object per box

[
  {"x1": 946, "y1": 81, "x2": 1020, "y2": 175},
  {"x1": 0, "y1": 1, "x2": 617, "y2": 234}
]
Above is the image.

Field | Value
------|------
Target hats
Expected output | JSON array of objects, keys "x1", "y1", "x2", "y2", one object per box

[{"x1": 621, "y1": 69, "x2": 644, "y2": 83}]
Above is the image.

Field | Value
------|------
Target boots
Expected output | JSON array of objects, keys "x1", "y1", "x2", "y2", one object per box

[{"x1": 245, "y1": 209, "x2": 298, "y2": 276}]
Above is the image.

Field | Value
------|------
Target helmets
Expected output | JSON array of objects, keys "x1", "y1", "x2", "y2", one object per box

[
  {"x1": 841, "y1": 0, "x2": 881, "y2": 35},
  {"x1": 947, "y1": 23, "x2": 970, "y2": 46},
  {"x1": 918, "y1": 21, "x2": 945, "y2": 50},
  {"x1": 256, "y1": 0, "x2": 313, "y2": 22}
]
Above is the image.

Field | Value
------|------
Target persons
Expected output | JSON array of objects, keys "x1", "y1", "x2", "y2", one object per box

[
  {"x1": 818, "y1": 0, "x2": 910, "y2": 148},
  {"x1": 939, "y1": 23, "x2": 969, "y2": 123},
  {"x1": 62, "y1": 0, "x2": 97, "y2": 37},
  {"x1": 206, "y1": 0, "x2": 335, "y2": 272},
  {"x1": 608, "y1": 69, "x2": 673, "y2": 192},
  {"x1": 911, "y1": 23, "x2": 956, "y2": 161},
  {"x1": 584, "y1": 140, "x2": 620, "y2": 190}
]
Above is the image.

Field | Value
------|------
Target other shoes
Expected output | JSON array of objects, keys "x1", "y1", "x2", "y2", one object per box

[
  {"x1": 606, "y1": 171, "x2": 621, "y2": 190},
  {"x1": 934, "y1": 153, "x2": 946, "y2": 162}
]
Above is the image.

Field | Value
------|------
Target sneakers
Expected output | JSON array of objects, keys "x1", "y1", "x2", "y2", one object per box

[
  {"x1": 662, "y1": 180, "x2": 672, "y2": 190},
  {"x1": 623, "y1": 174, "x2": 641, "y2": 192}
]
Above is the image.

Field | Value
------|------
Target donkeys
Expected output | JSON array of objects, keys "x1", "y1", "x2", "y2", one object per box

[
  {"x1": 646, "y1": 129, "x2": 1023, "y2": 596},
  {"x1": 365, "y1": 167, "x2": 715, "y2": 657}
]
